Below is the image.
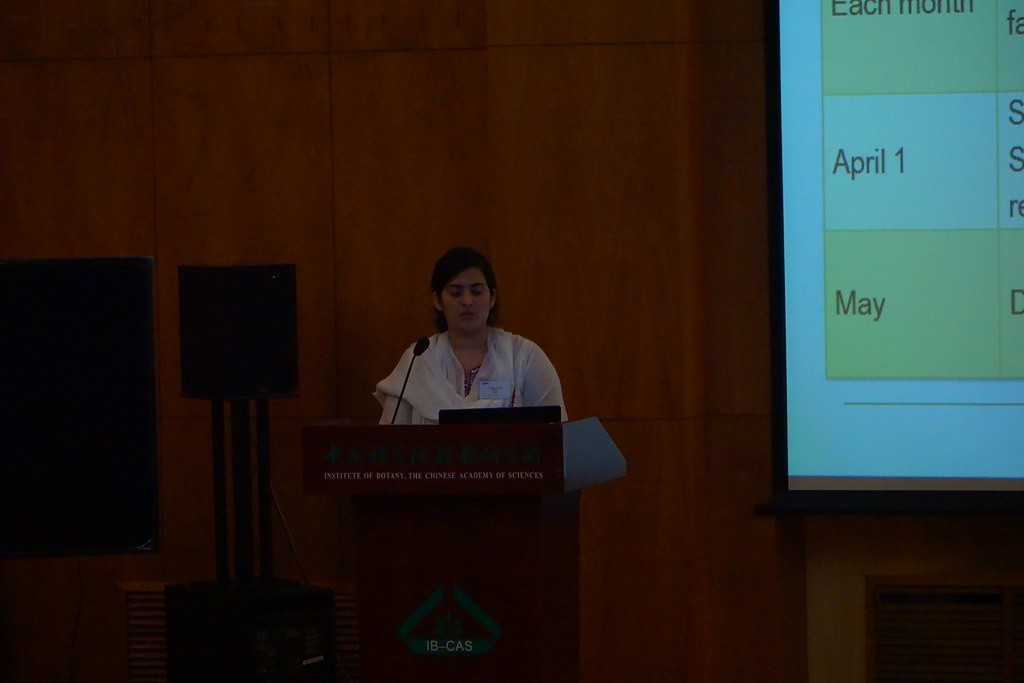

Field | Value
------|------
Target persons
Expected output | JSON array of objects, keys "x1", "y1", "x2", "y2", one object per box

[{"x1": 378, "y1": 247, "x2": 572, "y2": 429}]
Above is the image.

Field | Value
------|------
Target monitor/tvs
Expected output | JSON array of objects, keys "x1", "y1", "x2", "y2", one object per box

[{"x1": 0, "y1": 257, "x2": 162, "y2": 557}]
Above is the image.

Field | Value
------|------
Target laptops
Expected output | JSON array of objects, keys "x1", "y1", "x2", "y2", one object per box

[{"x1": 439, "y1": 405, "x2": 562, "y2": 425}]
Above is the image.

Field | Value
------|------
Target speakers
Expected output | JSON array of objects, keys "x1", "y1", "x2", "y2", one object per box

[
  {"x1": 178, "y1": 263, "x2": 298, "y2": 400},
  {"x1": 165, "y1": 577, "x2": 338, "y2": 683}
]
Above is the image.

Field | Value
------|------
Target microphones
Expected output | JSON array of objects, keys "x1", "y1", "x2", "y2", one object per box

[{"x1": 391, "y1": 337, "x2": 430, "y2": 424}]
